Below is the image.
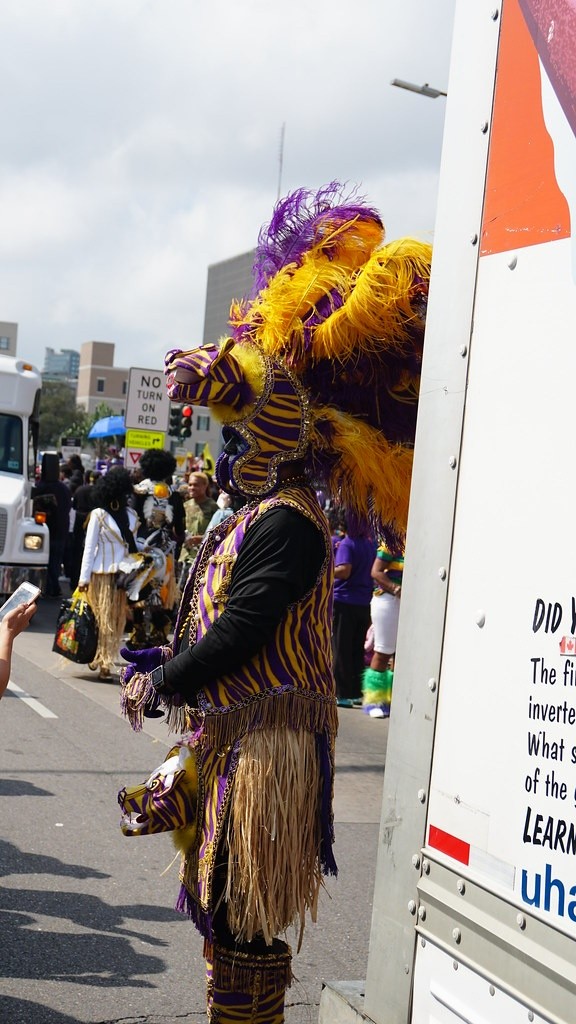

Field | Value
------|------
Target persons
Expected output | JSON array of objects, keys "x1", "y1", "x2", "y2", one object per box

[
  {"x1": 33, "y1": 449, "x2": 406, "y2": 720},
  {"x1": 0, "y1": 601, "x2": 37, "y2": 699},
  {"x1": 119, "y1": 427, "x2": 344, "y2": 1024}
]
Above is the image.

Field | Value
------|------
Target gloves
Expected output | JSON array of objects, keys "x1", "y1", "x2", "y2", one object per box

[
  {"x1": 120, "y1": 646, "x2": 161, "y2": 684},
  {"x1": 144, "y1": 702, "x2": 165, "y2": 718}
]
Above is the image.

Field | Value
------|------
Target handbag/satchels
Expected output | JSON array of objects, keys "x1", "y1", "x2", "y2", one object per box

[{"x1": 51, "y1": 587, "x2": 99, "y2": 664}]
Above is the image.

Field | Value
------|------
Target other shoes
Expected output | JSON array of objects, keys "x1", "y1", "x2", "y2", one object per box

[
  {"x1": 369, "y1": 707, "x2": 386, "y2": 717},
  {"x1": 98, "y1": 670, "x2": 113, "y2": 682},
  {"x1": 350, "y1": 697, "x2": 361, "y2": 704},
  {"x1": 88, "y1": 660, "x2": 98, "y2": 671},
  {"x1": 336, "y1": 698, "x2": 353, "y2": 707}
]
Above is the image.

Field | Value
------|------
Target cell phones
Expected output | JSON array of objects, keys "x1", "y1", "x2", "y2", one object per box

[{"x1": 0, "y1": 581, "x2": 42, "y2": 622}]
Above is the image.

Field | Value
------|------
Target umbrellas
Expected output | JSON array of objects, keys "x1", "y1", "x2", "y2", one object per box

[{"x1": 89, "y1": 414, "x2": 126, "y2": 445}]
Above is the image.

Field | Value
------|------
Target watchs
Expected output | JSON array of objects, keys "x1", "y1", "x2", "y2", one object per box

[{"x1": 151, "y1": 665, "x2": 167, "y2": 695}]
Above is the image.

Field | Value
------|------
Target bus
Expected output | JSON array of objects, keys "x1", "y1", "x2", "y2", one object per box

[{"x1": 0, "y1": 354, "x2": 49, "y2": 605}]
